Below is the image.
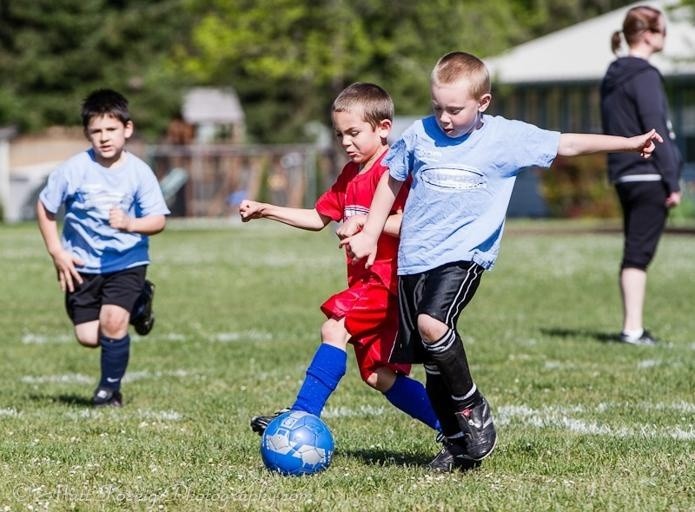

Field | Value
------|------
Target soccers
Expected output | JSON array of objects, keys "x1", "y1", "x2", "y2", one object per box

[{"x1": 260, "y1": 410, "x2": 334, "y2": 476}]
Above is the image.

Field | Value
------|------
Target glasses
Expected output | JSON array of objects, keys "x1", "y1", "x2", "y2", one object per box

[{"x1": 650, "y1": 27, "x2": 666, "y2": 37}]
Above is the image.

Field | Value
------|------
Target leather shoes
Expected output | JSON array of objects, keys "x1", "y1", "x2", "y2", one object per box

[{"x1": 250, "y1": 408, "x2": 289, "y2": 436}]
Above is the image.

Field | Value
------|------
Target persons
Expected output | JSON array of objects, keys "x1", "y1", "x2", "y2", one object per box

[
  {"x1": 600, "y1": 6, "x2": 684, "y2": 348},
  {"x1": 36, "y1": 88, "x2": 170, "y2": 406},
  {"x1": 239, "y1": 52, "x2": 664, "y2": 475}
]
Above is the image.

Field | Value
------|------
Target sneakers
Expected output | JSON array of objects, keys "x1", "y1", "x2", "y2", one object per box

[
  {"x1": 92, "y1": 391, "x2": 122, "y2": 408},
  {"x1": 619, "y1": 329, "x2": 654, "y2": 346},
  {"x1": 424, "y1": 396, "x2": 498, "y2": 471},
  {"x1": 130, "y1": 280, "x2": 154, "y2": 336}
]
